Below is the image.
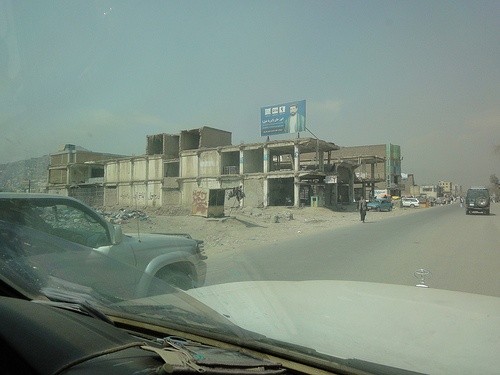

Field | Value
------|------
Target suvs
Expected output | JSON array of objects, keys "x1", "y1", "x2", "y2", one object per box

[
  {"x1": 401, "y1": 198, "x2": 420, "y2": 208},
  {"x1": 0, "y1": 193, "x2": 207, "y2": 305},
  {"x1": 466, "y1": 187, "x2": 490, "y2": 215}
]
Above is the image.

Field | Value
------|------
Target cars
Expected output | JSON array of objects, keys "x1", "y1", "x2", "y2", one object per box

[{"x1": 367, "y1": 198, "x2": 393, "y2": 212}]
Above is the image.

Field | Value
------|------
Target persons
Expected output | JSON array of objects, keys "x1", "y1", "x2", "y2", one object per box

[
  {"x1": 282, "y1": 103, "x2": 305, "y2": 133},
  {"x1": 356, "y1": 195, "x2": 369, "y2": 224}
]
